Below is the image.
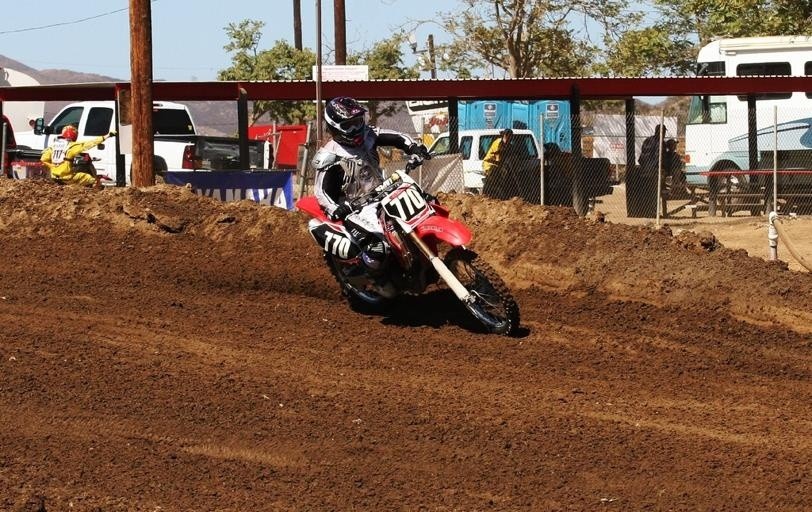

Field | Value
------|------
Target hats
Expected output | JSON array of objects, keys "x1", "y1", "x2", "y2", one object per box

[{"x1": 655, "y1": 125, "x2": 666, "y2": 132}]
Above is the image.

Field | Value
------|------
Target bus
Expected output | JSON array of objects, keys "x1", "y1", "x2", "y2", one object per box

[{"x1": 683, "y1": 34, "x2": 812, "y2": 215}]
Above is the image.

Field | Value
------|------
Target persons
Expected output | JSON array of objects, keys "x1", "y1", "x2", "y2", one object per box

[
  {"x1": 40, "y1": 126, "x2": 118, "y2": 188},
  {"x1": 314, "y1": 97, "x2": 425, "y2": 298},
  {"x1": 483, "y1": 128, "x2": 513, "y2": 174},
  {"x1": 639, "y1": 124, "x2": 691, "y2": 197}
]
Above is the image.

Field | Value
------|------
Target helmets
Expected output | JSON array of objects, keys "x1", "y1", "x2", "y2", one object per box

[
  {"x1": 62, "y1": 125, "x2": 79, "y2": 141},
  {"x1": 324, "y1": 98, "x2": 368, "y2": 147}
]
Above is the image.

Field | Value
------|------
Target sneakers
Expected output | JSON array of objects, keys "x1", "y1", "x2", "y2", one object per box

[{"x1": 367, "y1": 278, "x2": 395, "y2": 298}]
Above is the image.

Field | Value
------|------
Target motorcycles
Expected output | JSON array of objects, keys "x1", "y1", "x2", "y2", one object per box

[
  {"x1": 10, "y1": 152, "x2": 102, "y2": 188},
  {"x1": 295, "y1": 138, "x2": 521, "y2": 338}
]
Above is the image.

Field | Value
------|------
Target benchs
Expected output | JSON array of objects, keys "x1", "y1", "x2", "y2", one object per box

[
  {"x1": 694, "y1": 203, "x2": 812, "y2": 219},
  {"x1": 694, "y1": 190, "x2": 812, "y2": 204}
]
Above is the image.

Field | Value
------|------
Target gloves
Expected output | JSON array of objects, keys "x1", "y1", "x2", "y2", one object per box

[
  {"x1": 333, "y1": 205, "x2": 351, "y2": 218},
  {"x1": 408, "y1": 142, "x2": 429, "y2": 160}
]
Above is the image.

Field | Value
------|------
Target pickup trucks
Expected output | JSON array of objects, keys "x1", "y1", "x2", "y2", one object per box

[
  {"x1": 33, "y1": 99, "x2": 272, "y2": 189},
  {"x1": 428, "y1": 129, "x2": 613, "y2": 197}
]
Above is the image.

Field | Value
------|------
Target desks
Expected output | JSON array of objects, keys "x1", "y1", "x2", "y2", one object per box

[{"x1": 700, "y1": 170, "x2": 811, "y2": 218}]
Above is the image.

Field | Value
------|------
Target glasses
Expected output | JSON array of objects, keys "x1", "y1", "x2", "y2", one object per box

[{"x1": 324, "y1": 107, "x2": 365, "y2": 135}]
